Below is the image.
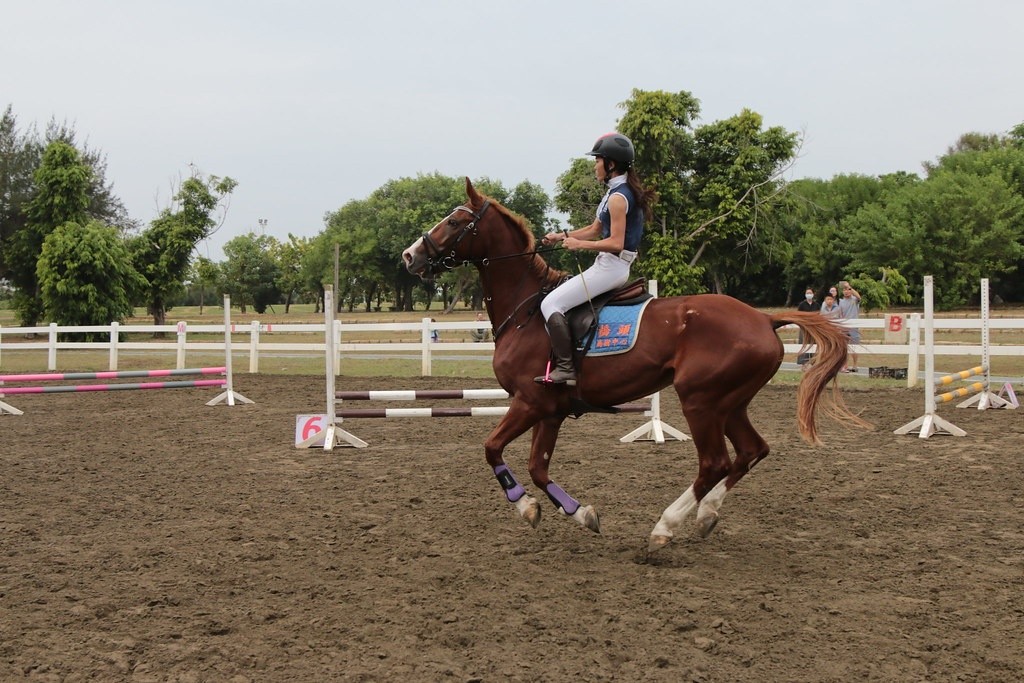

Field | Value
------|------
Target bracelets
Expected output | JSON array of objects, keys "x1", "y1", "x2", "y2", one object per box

[{"x1": 849, "y1": 288, "x2": 852, "y2": 290}]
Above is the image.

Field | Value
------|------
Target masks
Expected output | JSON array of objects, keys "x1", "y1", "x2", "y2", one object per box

[{"x1": 806, "y1": 294, "x2": 814, "y2": 299}]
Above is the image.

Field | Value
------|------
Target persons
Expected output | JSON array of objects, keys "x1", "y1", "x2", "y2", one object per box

[
  {"x1": 838, "y1": 281, "x2": 861, "y2": 371},
  {"x1": 820, "y1": 287, "x2": 850, "y2": 372},
  {"x1": 469, "y1": 313, "x2": 489, "y2": 343},
  {"x1": 431, "y1": 319, "x2": 438, "y2": 343},
  {"x1": 534, "y1": 134, "x2": 656, "y2": 386},
  {"x1": 797, "y1": 287, "x2": 819, "y2": 363}
]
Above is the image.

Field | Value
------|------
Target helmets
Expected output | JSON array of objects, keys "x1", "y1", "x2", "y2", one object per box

[{"x1": 585, "y1": 132, "x2": 635, "y2": 163}]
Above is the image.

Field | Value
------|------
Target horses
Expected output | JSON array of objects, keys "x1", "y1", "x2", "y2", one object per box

[{"x1": 400, "y1": 177, "x2": 864, "y2": 552}]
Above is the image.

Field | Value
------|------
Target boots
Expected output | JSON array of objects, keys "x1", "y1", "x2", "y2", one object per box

[{"x1": 534, "y1": 312, "x2": 577, "y2": 386}]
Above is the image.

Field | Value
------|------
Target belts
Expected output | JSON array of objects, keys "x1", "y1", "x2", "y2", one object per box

[{"x1": 609, "y1": 252, "x2": 633, "y2": 263}]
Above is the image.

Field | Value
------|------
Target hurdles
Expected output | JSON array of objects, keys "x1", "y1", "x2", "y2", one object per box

[
  {"x1": 0, "y1": 292, "x2": 257, "y2": 417},
  {"x1": 893, "y1": 272, "x2": 1018, "y2": 441},
  {"x1": 293, "y1": 278, "x2": 693, "y2": 454}
]
